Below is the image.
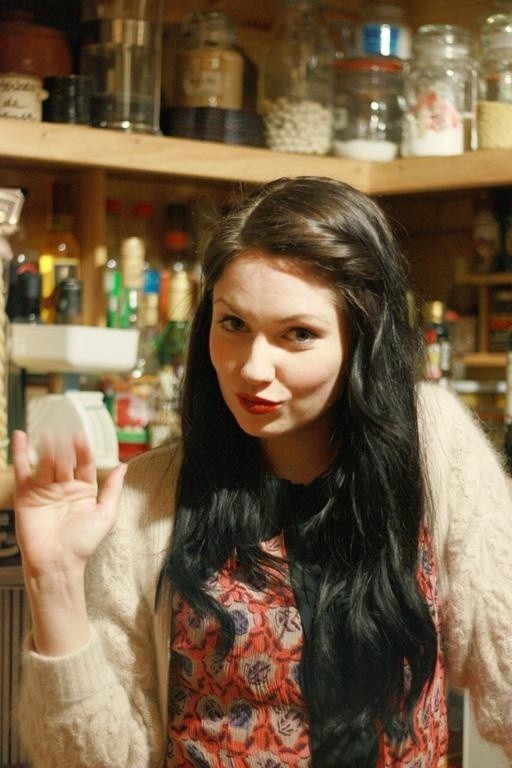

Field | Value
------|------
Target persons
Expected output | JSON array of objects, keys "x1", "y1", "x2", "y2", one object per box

[{"x1": 10, "y1": 174, "x2": 510, "y2": 767}]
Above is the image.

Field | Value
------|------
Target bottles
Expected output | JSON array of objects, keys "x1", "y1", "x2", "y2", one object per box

[
  {"x1": 161, "y1": 11, "x2": 258, "y2": 148},
  {"x1": 8, "y1": 196, "x2": 512, "y2": 450},
  {"x1": 476, "y1": 13, "x2": 512, "y2": 150},
  {"x1": 260, "y1": 0, "x2": 337, "y2": 156},
  {"x1": 331, "y1": 57, "x2": 404, "y2": 163},
  {"x1": 399, "y1": 23, "x2": 486, "y2": 159}
]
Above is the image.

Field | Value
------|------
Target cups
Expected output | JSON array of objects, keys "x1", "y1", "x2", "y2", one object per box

[
  {"x1": 0, "y1": 71, "x2": 51, "y2": 122},
  {"x1": 43, "y1": 74, "x2": 96, "y2": 127}
]
